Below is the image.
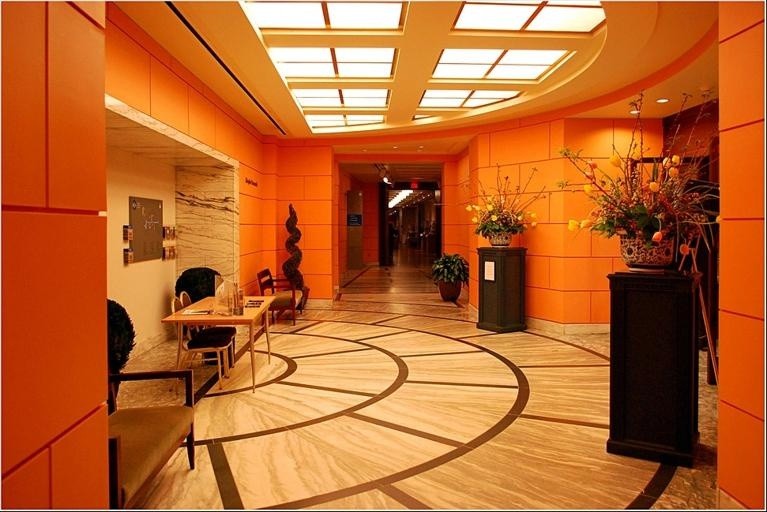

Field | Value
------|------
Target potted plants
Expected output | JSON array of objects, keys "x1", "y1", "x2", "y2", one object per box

[
  {"x1": 283, "y1": 202, "x2": 310, "y2": 311},
  {"x1": 431, "y1": 254, "x2": 469, "y2": 302},
  {"x1": 108, "y1": 299, "x2": 137, "y2": 402}
]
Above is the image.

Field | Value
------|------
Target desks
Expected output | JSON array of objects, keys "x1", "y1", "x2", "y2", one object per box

[{"x1": 160, "y1": 296, "x2": 277, "y2": 394}]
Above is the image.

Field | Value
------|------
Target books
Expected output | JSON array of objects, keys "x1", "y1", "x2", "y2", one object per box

[{"x1": 182, "y1": 310, "x2": 210, "y2": 315}]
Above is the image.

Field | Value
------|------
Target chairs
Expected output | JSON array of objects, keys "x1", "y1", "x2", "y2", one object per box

[
  {"x1": 108, "y1": 369, "x2": 196, "y2": 509},
  {"x1": 170, "y1": 290, "x2": 237, "y2": 390},
  {"x1": 256, "y1": 269, "x2": 303, "y2": 326}
]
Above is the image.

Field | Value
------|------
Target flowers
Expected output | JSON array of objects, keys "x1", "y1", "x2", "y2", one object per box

[
  {"x1": 465, "y1": 163, "x2": 552, "y2": 239},
  {"x1": 553, "y1": 85, "x2": 721, "y2": 257}
]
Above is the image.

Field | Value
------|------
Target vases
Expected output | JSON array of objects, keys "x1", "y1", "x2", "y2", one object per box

[
  {"x1": 485, "y1": 231, "x2": 513, "y2": 248},
  {"x1": 616, "y1": 226, "x2": 676, "y2": 274}
]
